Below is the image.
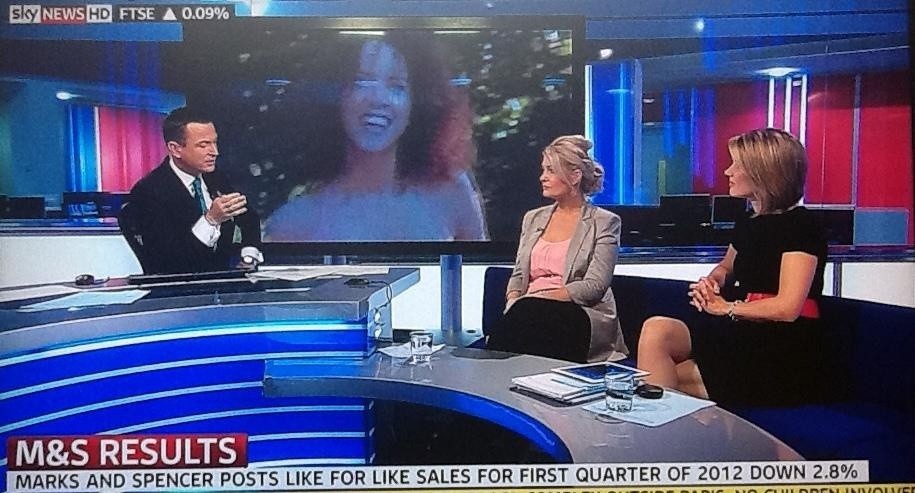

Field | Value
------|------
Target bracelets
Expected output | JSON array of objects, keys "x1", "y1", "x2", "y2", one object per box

[{"x1": 727, "y1": 299, "x2": 746, "y2": 320}]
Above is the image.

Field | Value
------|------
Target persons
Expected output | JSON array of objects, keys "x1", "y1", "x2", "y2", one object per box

[
  {"x1": 637, "y1": 126, "x2": 826, "y2": 409},
  {"x1": 263, "y1": 32, "x2": 489, "y2": 241},
  {"x1": 480, "y1": 134, "x2": 630, "y2": 364},
  {"x1": 128, "y1": 106, "x2": 263, "y2": 278}
]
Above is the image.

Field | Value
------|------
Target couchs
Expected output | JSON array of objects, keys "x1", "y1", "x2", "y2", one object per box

[{"x1": 468, "y1": 268, "x2": 915, "y2": 482}]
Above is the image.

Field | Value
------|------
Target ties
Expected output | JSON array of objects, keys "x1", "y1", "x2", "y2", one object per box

[{"x1": 192, "y1": 178, "x2": 207, "y2": 214}]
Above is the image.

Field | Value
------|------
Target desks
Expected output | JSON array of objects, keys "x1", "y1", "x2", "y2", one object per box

[{"x1": 3, "y1": 264, "x2": 809, "y2": 493}]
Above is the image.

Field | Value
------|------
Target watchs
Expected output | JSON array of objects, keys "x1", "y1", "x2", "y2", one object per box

[{"x1": 241, "y1": 254, "x2": 257, "y2": 265}]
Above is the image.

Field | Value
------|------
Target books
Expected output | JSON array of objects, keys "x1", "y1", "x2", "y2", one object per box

[{"x1": 511, "y1": 359, "x2": 650, "y2": 404}]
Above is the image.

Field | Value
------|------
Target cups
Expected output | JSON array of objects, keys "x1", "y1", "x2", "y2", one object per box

[
  {"x1": 604, "y1": 372, "x2": 633, "y2": 413},
  {"x1": 410, "y1": 330, "x2": 433, "y2": 363}
]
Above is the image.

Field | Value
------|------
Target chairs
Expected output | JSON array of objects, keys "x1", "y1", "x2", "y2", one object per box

[{"x1": 120, "y1": 202, "x2": 150, "y2": 275}]
